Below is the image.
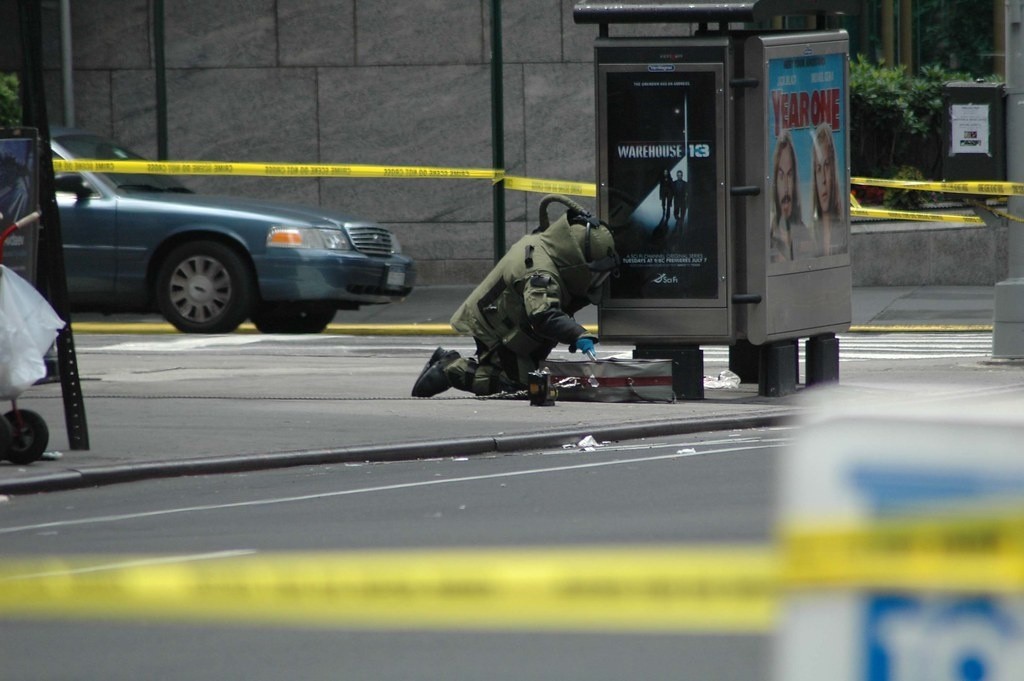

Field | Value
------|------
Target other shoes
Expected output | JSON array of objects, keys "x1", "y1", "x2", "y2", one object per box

[{"x1": 411, "y1": 347, "x2": 460, "y2": 397}]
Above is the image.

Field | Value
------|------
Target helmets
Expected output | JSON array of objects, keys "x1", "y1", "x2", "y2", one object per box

[{"x1": 571, "y1": 222, "x2": 620, "y2": 271}]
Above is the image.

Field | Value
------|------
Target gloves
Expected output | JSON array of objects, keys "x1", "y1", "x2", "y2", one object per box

[{"x1": 576, "y1": 338, "x2": 596, "y2": 356}]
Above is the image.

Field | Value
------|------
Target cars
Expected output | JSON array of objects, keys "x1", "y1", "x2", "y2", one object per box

[{"x1": 0, "y1": 124, "x2": 418, "y2": 337}]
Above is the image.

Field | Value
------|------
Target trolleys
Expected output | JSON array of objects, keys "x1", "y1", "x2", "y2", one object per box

[{"x1": 2, "y1": 212, "x2": 50, "y2": 467}]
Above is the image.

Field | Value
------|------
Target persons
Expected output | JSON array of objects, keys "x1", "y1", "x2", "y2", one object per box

[
  {"x1": 409, "y1": 217, "x2": 617, "y2": 396},
  {"x1": 811, "y1": 122, "x2": 847, "y2": 261},
  {"x1": 767, "y1": 130, "x2": 818, "y2": 265},
  {"x1": 659, "y1": 167, "x2": 688, "y2": 232}
]
